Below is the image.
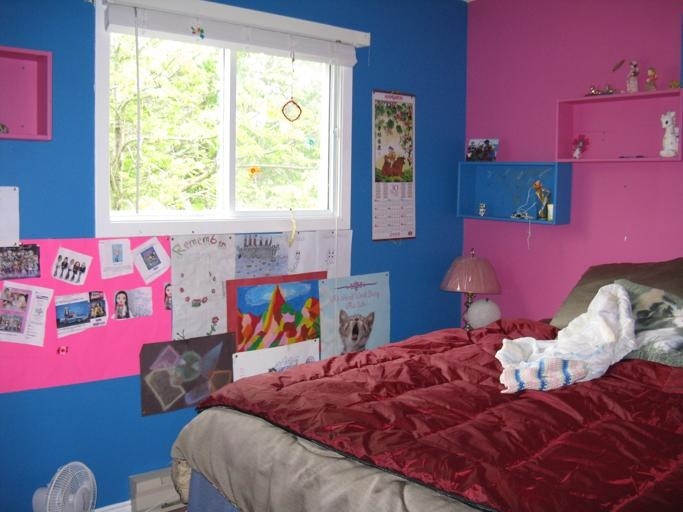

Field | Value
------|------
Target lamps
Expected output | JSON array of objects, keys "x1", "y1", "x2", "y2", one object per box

[{"x1": 440, "y1": 247, "x2": 500, "y2": 331}]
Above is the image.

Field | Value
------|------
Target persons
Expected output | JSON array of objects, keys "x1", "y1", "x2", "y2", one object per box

[
  {"x1": 532, "y1": 179, "x2": 552, "y2": 218},
  {"x1": 53, "y1": 255, "x2": 86, "y2": 283},
  {"x1": 164, "y1": 284, "x2": 172, "y2": 310},
  {"x1": 613, "y1": 59, "x2": 640, "y2": 92},
  {"x1": 468, "y1": 140, "x2": 492, "y2": 159},
  {"x1": 112, "y1": 290, "x2": 133, "y2": 319}
]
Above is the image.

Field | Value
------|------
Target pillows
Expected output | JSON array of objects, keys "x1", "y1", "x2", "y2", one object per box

[{"x1": 549, "y1": 254, "x2": 683, "y2": 331}]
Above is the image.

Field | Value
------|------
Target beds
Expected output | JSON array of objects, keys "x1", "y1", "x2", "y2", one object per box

[{"x1": 185, "y1": 318, "x2": 683, "y2": 511}]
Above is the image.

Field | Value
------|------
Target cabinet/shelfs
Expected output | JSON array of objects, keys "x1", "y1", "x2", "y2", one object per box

[{"x1": 454, "y1": 89, "x2": 682, "y2": 226}]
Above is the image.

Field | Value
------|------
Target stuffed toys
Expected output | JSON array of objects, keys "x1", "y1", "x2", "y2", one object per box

[{"x1": 658, "y1": 112, "x2": 679, "y2": 157}]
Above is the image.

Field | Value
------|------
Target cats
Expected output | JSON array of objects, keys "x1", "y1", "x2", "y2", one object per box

[{"x1": 338, "y1": 309, "x2": 376, "y2": 357}]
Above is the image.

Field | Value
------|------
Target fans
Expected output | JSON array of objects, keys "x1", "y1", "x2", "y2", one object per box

[{"x1": 31, "y1": 459, "x2": 97, "y2": 511}]
